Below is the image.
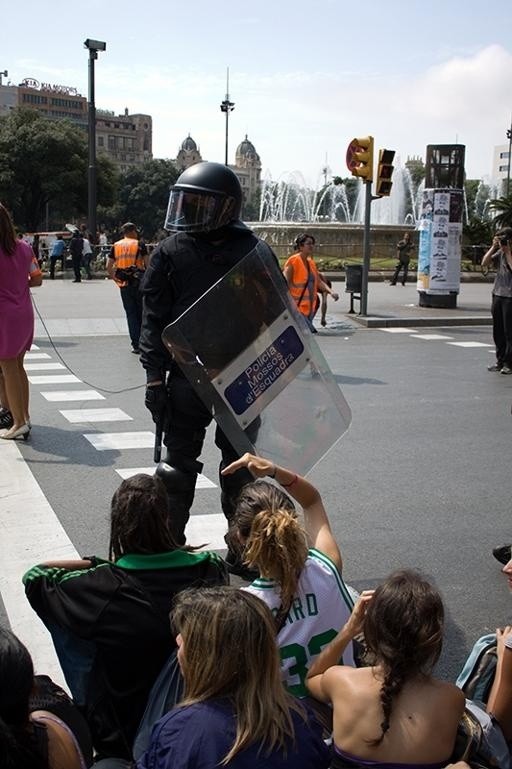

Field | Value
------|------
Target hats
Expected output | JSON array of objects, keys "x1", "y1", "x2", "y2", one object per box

[{"x1": 122, "y1": 222, "x2": 141, "y2": 233}]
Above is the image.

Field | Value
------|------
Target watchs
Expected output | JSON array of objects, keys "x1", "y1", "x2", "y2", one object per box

[{"x1": 84, "y1": 555, "x2": 94, "y2": 566}]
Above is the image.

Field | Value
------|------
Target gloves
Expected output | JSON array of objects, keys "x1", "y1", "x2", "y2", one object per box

[{"x1": 145, "y1": 385, "x2": 178, "y2": 431}]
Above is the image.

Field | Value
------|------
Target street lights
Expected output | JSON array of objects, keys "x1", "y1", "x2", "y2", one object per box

[{"x1": 85, "y1": 37, "x2": 107, "y2": 262}]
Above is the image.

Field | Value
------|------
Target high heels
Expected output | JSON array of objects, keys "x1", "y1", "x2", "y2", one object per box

[
  {"x1": 25, "y1": 419, "x2": 33, "y2": 431},
  {"x1": 0, "y1": 427, "x2": 30, "y2": 440}
]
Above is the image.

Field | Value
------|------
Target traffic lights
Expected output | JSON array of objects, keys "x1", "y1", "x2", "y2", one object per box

[
  {"x1": 375, "y1": 150, "x2": 395, "y2": 198},
  {"x1": 347, "y1": 139, "x2": 374, "y2": 185}
]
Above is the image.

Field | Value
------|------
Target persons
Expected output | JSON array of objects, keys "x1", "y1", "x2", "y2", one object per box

[
  {"x1": 480, "y1": 227, "x2": 512, "y2": 375},
  {"x1": 49, "y1": 235, "x2": 67, "y2": 278},
  {"x1": 0, "y1": 369, "x2": 14, "y2": 429},
  {"x1": 223, "y1": 451, "x2": 361, "y2": 697},
  {"x1": 452, "y1": 540, "x2": 512, "y2": 745},
  {"x1": 140, "y1": 162, "x2": 286, "y2": 581},
  {"x1": 71, "y1": 230, "x2": 83, "y2": 281},
  {"x1": 284, "y1": 234, "x2": 339, "y2": 378},
  {"x1": 0, "y1": 201, "x2": 42, "y2": 439},
  {"x1": 0, "y1": 628, "x2": 95, "y2": 769},
  {"x1": 144, "y1": 586, "x2": 334, "y2": 769},
  {"x1": 105, "y1": 223, "x2": 150, "y2": 353},
  {"x1": 390, "y1": 232, "x2": 415, "y2": 286},
  {"x1": 82, "y1": 238, "x2": 93, "y2": 280},
  {"x1": 303, "y1": 569, "x2": 512, "y2": 768},
  {"x1": 316, "y1": 271, "x2": 332, "y2": 326},
  {"x1": 30, "y1": 235, "x2": 43, "y2": 269},
  {"x1": 81, "y1": 225, "x2": 112, "y2": 242},
  {"x1": 21, "y1": 475, "x2": 229, "y2": 760}
]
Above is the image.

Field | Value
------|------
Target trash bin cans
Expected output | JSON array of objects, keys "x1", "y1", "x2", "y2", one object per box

[{"x1": 344, "y1": 264, "x2": 363, "y2": 294}]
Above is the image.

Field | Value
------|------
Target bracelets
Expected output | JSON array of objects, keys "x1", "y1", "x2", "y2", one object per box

[{"x1": 283, "y1": 475, "x2": 299, "y2": 488}]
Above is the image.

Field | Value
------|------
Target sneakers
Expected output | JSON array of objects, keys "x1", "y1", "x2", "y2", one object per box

[
  {"x1": 499, "y1": 364, "x2": 512, "y2": 375},
  {"x1": 1, "y1": 411, "x2": 13, "y2": 428},
  {"x1": 487, "y1": 362, "x2": 501, "y2": 371}
]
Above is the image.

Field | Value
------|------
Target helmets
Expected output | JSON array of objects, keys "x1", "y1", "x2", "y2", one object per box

[{"x1": 163, "y1": 163, "x2": 243, "y2": 233}]
